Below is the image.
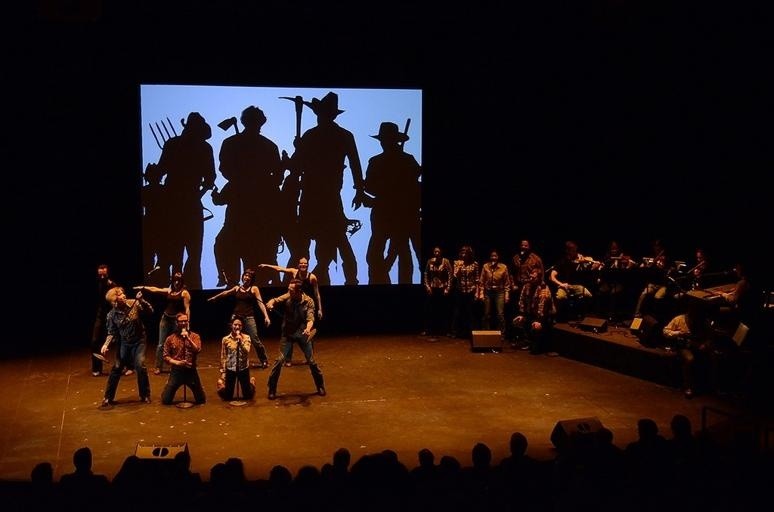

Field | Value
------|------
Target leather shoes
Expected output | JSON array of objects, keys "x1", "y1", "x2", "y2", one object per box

[
  {"x1": 93, "y1": 370, "x2": 98, "y2": 376},
  {"x1": 140, "y1": 395, "x2": 151, "y2": 404},
  {"x1": 269, "y1": 393, "x2": 274, "y2": 400},
  {"x1": 319, "y1": 387, "x2": 326, "y2": 395},
  {"x1": 102, "y1": 398, "x2": 110, "y2": 406},
  {"x1": 125, "y1": 369, "x2": 133, "y2": 376},
  {"x1": 287, "y1": 362, "x2": 291, "y2": 367}
]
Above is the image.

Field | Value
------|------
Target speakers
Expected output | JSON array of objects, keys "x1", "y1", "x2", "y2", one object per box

[
  {"x1": 470, "y1": 330, "x2": 501, "y2": 348},
  {"x1": 134, "y1": 443, "x2": 189, "y2": 471},
  {"x1": 579, "y1": 316, "x2": 608, "y2": 333},
  {"x1": 550, "y1": 416, "x2": 605, "y2": 453}
]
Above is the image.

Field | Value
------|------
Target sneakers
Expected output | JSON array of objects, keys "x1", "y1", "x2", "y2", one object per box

[{"x1": 154, "y1": 370, "x2": 160, "y2": 375}]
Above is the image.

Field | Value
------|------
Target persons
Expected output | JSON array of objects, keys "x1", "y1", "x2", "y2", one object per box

[
  {"x1": 422, "y1": 237, "x2": 712, "y2": 355},
  {"x1": 89, "y1": 255, "x2": 329, "y2": 407},
  {"x1": 0, "y1": 415, "x2": 774, "y2": 511},
  {"x1": 719, "y1": 256, "x2": 773, "y2": 388}
]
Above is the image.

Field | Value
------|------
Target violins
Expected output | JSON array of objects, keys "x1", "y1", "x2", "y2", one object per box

[
  {"x1": 693, "y1": 265, "x2": 704, "y2": 278},
  {"x1": 648, "y1": 257, "x2": 664, "y2": 267},
  {"x1": 574, "y1": 257, "x2": 604, "y2": 270},
  {"x1": 620, "y1": 254, "x2": 636, "y2": 264}
]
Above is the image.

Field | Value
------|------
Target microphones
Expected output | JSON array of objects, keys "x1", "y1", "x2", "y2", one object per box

[
  {"x1": 491, "y1": 261, "x2": 494, "y2": 268},
  {"x1": 235, "y1": 330, "x2": 240, "y2": 343},
  {"x1": 544, "y1": 265, "x2": 554, "y2": 273},
  {"x1": 519, "y1": 251, "x2": 524, "y2": 260},
  {"x1": 181, "y1": 328, "x2": 186, "y2": 338},
  {"x1": 147, "y1": 266, "x2": 160, "y2": 275},
  {"x1": 717, "y1": 271, "x2": 728, "y2": 276},
  {"x1": 220, "y1": 268, "x2": 229, "y2": 284}
]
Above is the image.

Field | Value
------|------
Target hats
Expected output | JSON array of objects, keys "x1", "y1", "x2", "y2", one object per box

[
  {"x1": 143, "y1": 163, "x2": 159, "y2": 176},
  {"x1": 180, "y1": 112, "x2": 204, "y2": 127},
  {"x1": 369, "y1": 122, "x2": 409, "y2": 142},
  {"x1": 312, "y1": 91, "x2": 344, "y2": 116}
]
